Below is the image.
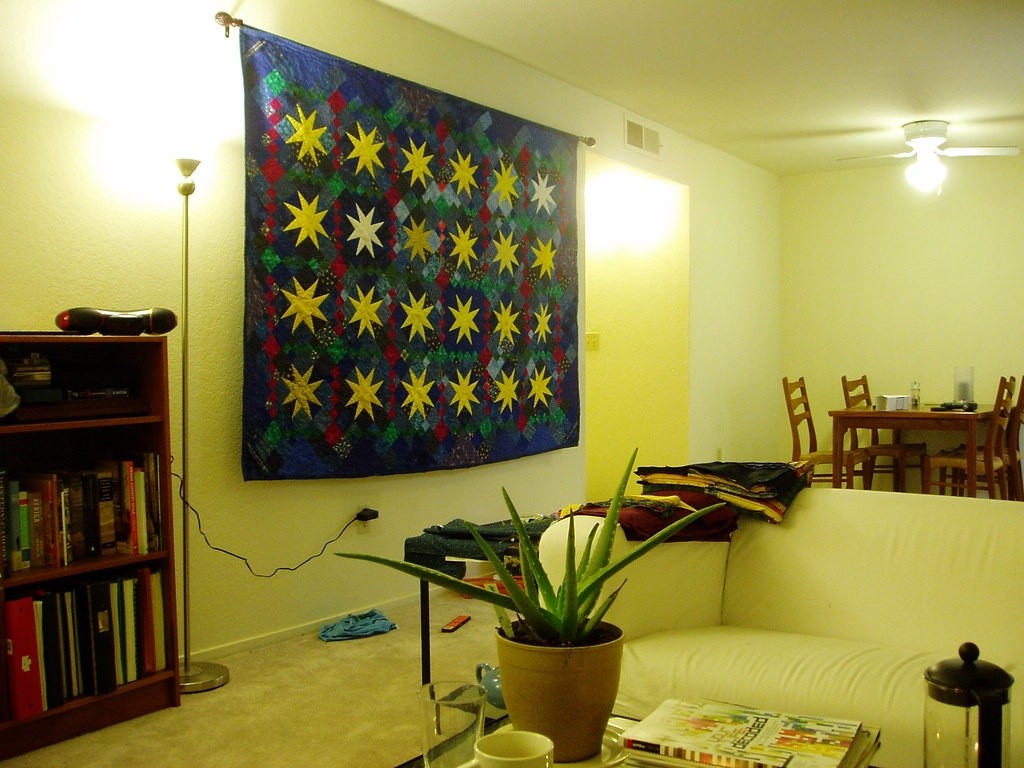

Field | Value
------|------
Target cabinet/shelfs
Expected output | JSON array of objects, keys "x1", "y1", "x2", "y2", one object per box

[{"x1": 0, "y1": 331, "x2": 181, "y2": 761}]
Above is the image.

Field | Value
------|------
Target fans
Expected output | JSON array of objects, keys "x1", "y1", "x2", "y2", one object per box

[{"x1": 835, "y1": 120, "x2": 1019, "y2": 163}]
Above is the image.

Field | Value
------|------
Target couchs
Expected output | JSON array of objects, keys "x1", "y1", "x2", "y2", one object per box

[{"x1": 539, "y1": 488, "x2": 1024, "y2": 768}]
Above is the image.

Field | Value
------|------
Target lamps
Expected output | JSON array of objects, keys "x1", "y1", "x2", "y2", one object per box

[{"x1": 172, "y1": 160, "x2": 229, "y2": 694}]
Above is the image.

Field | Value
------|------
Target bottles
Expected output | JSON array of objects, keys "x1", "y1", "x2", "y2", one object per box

[
  {"x1": 910, "y1": 382, "x2": 920, "y2": 406},
  {"x1": 536, "y1": 514, "x2": 547, "y2": 519}
]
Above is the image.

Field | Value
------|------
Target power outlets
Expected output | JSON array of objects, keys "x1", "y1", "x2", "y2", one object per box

[{"x1": 356, "y1": 504, "x2": 370, "y2": 534}]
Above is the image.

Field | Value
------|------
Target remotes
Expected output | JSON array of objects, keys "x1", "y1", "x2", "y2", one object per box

[{"x1": 442, "y1": 615, "x2": 472, "y2": 632}]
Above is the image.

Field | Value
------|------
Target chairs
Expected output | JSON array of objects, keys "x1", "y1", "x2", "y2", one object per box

[
  {"x1": 952, "y1": 374, "x2": 1024, "y2": 501},
  {"x1": 782, "y1": 376, "x2": 870, "y2": 490},
  {"x1": 924, "y1": 376, "x2": 1016, "y2": 501},
  {"x1": 842, "y1": 375, "x2": 927, "y2": 494}
]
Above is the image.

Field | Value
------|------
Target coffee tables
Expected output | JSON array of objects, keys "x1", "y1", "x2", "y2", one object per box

[{"x1": 393, "y1": 714, "x2": 641, "y2": 768}]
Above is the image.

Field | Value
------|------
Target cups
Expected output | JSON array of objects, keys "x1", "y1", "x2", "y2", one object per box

[
  {"x1": 476, "y1": 664, "x2": 505, "y2": 708},
  {"x1": 954, "y1": 367, "x2": 974, "y2": 403},
  {"x1": 923, "y1": 642, "x2": 1015, "y2": 768},
  {"x1": 417, "y1": 681, "x2": 487, "y2": 768},
  {"x1": 475, "y1": 730, "x2": 554, "y2": 768}
]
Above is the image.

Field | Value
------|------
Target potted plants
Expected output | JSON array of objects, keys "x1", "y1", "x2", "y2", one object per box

[{"x1": 333, "y1": 446, "x2": 726, "y2": 763}]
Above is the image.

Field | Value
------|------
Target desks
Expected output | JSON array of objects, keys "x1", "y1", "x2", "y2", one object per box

[
  {"x1": 827, "y1": 403, "x2": 1015, "y2": 498},
  {"x1": 404, "y1": 516, "x2": 551, "y2": 688}
]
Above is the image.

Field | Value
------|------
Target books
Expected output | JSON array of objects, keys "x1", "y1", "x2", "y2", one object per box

[
  {"x1": 618, "y1": 698, "x2": 880, "y2": 768},
  {"x1": 0, "y1": 452, "x2": 168, "y2": 721}
]
